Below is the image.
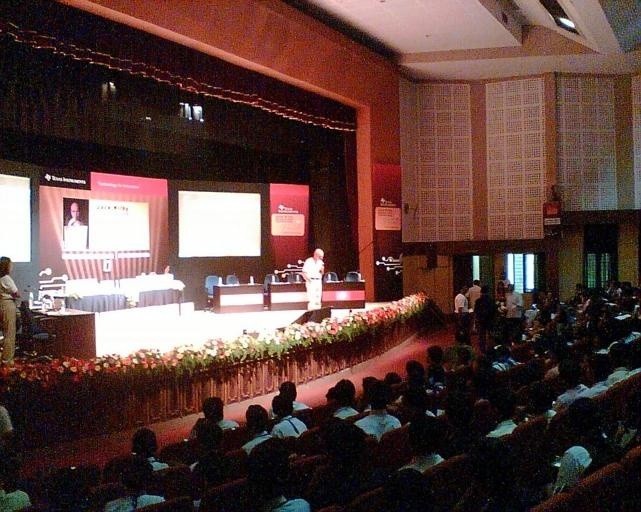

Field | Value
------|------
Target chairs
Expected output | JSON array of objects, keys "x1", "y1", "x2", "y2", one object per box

[
  {"x1": 200, "y1": 274, "x2": 280, "y2": 311},
  {"x1": 287, "y1": 271, "x2": 359, "y2": 283},
  {"x1": 17, "y1": 301, "x2": 61, "y2": 363},
  {"x1": 20, "y1": 296, "x2": 640, "y2": 512}
]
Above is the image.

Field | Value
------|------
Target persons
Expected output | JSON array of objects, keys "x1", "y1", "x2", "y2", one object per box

[{"x1": 65, "y1": 202, "x2": 86, "y2": 227}]
